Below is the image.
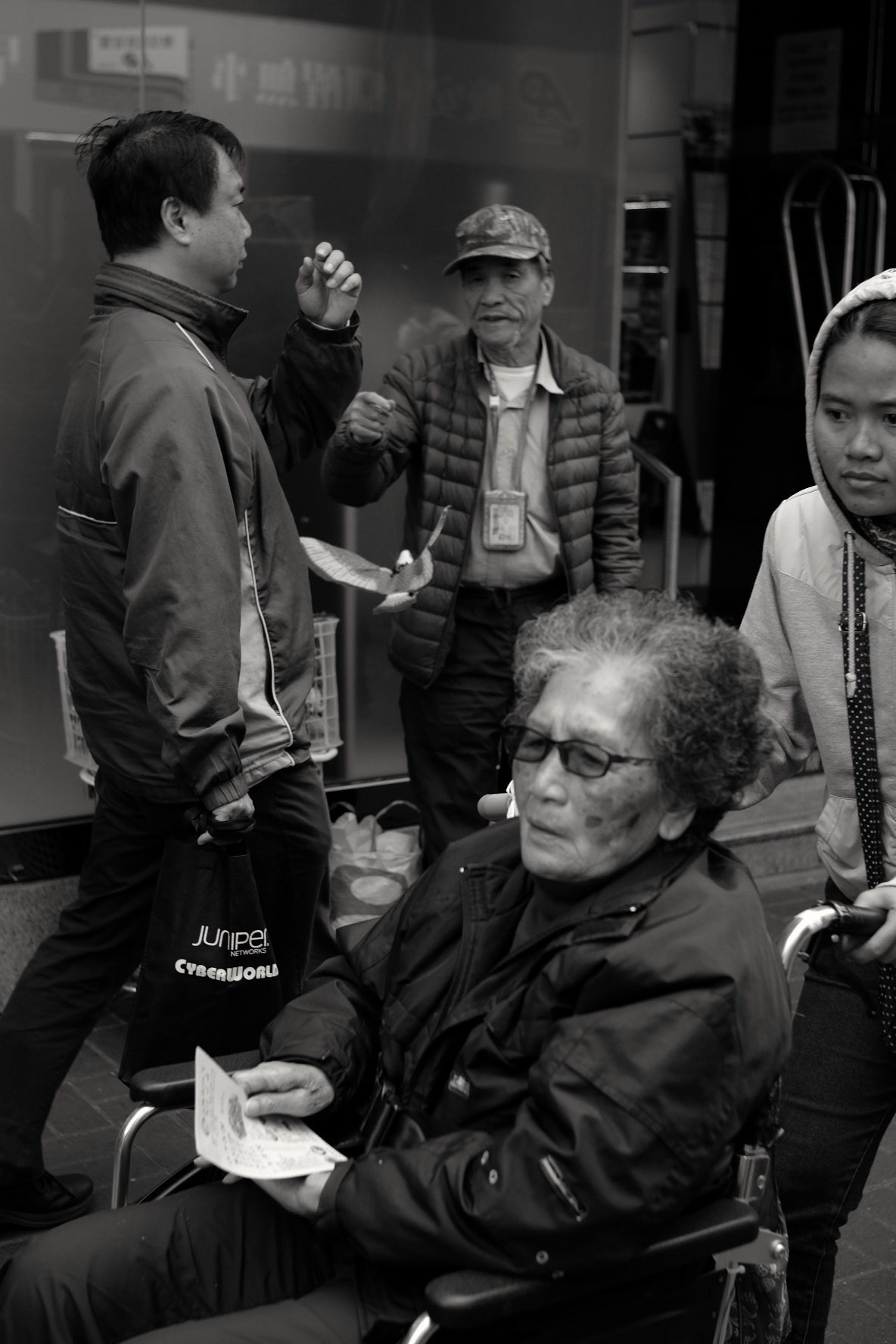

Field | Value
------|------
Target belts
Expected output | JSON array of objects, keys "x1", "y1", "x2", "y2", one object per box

[{"x1": 455, "y1": 579, "x2": 568, "y2": 611}]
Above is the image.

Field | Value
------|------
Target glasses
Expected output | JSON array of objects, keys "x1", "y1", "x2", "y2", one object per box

[{"x1": 498, "y1": 720, "x2": 694, "y2": 779}]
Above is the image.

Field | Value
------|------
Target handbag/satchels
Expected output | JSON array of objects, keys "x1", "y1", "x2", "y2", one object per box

[
  {"x1": 116, "y1": 808, "x2": 302, "y2": 1087},
  {"x1": 321, "y1": 800, "x2": 423, "y2": 955}
]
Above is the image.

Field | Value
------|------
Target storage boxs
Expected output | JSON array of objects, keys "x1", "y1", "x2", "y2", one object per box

[{"x1": 45, "y1": 613, "x2": 352, "y2": 785}]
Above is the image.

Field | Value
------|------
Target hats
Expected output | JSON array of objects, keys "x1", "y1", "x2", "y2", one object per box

[{"x1": 443, "y1": 205, "x2": 551, "y2": 274}]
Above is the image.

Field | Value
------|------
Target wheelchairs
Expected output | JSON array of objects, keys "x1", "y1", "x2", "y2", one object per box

[{"x1": 1, "y1": 791, "x2": 886, "y2": 1344}]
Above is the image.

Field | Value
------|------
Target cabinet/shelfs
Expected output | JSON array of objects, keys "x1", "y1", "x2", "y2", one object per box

[{"x1": 615, "y1": 196, "x2": 685, "y2": 412}]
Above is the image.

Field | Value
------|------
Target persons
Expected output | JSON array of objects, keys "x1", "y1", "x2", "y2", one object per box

[
  {"x1": 1, "y1": 586, "x2": 786, "y2": 1344},
  {"x1": 1, "y1": 113, "x2": 367, "y2": 1236},
  {"x1": 472, "y1": 271, "x2": 896, "y2": 1343},
  {"x1": 322, "y1": 206, "x2": 644, "y2": 878}
]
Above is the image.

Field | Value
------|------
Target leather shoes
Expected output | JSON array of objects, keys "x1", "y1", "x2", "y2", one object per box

[{"x1": 0, "y1": 1172, "x2": 93, "y2": 1241}]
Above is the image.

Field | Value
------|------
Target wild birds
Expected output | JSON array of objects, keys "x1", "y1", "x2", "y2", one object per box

[{"x1": 299, "y1": 503, "x2": 450, "y2": 614}]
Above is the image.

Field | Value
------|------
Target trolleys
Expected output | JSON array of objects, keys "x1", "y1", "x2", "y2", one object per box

[{"x1": 50, "y1": 615, "x2": 343, "y2": 836}]
